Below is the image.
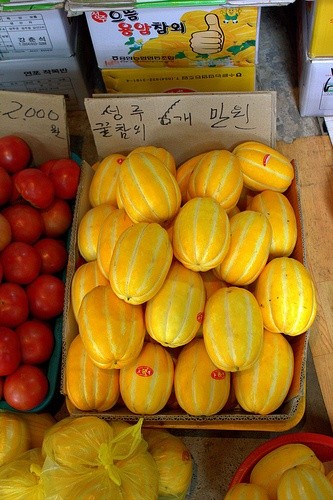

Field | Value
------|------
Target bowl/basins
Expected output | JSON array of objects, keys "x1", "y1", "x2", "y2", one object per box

[{"x1": 231, "y1": 433, "x2": 333, "y2": 490}]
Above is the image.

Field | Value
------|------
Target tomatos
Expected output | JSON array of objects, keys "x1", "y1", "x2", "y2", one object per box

[{"x1": 0, "y1": 134, "x2": 81, "y2": 410}]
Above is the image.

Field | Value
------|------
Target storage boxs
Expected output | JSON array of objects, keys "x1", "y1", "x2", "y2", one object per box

[{"x1": 0, "y1": 0, "x2": 333, "y2": 430}]
